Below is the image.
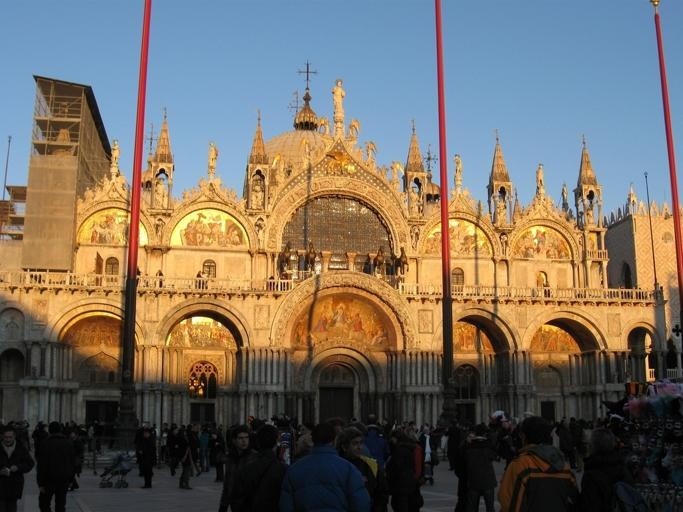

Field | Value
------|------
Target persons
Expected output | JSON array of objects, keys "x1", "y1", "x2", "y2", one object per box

[
  {"x1": 201, "y1": 272, "x2": 207, "y2": 288},
  {"x1": 1, "y1": 410, "x2": 682, "y2": 511},
  {"x1": 607, "y1": 285, "x2": 649, "y2": 302},
  {"x1": 156, "y1": 269, "x2": 163, "y2": 288},
  {"x1": 534, "y1": 163, "x2": 545, "y2": 189},
  {"x1": 279, "y1": 274, "x2": 287, "y2": 291},
  {"x1": 109, "y1": 139, "x2": 120, "y2": 169},
  {"x1": 268, "y1": 275, "x2": 274, "y2": 291},
  {"x1": 543, "y1": 281, "x2": 552, "y2": 298},
  {"x1": 451, "y1": 154, "x2": 463, "y2": 180},
  {"x1": 331, "y1": 77, "x2": 346, "y2": 116},
  {"x1": 195, "y1": 271, "x2": 199, "y2": 288},
  {"x1": 206, "y1": 142, "x2": 219, "y2": 170},
  {"x1": 132, "y1": 265, "x2": 141, "y2": 287}
]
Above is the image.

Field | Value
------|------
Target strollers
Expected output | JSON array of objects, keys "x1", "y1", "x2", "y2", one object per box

[{"x1": 98, "y1": 452, "x2": 135, "y2": 490}]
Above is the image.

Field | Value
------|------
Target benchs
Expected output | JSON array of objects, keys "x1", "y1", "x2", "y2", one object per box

[{"x1": 79, "y1": 450, "x2": 137, "y2": 475}]
